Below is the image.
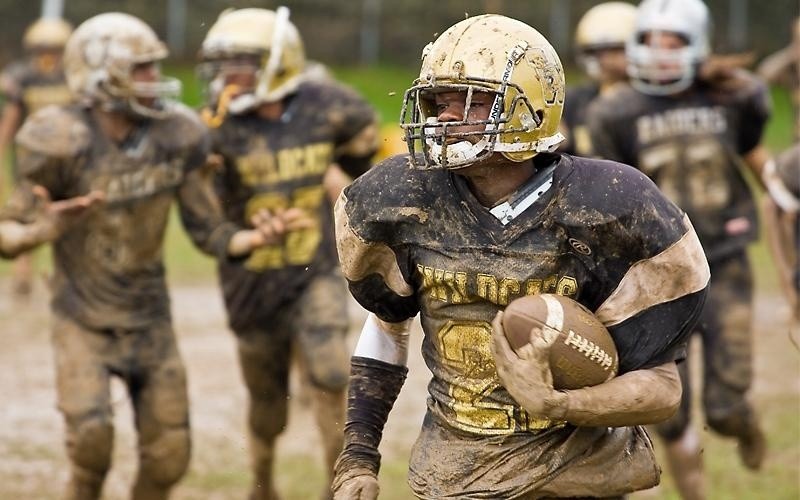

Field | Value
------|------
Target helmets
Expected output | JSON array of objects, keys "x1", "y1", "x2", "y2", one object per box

[
  {"x1": 400, "y1": 14, "x2": 565, "y2": 170},
  {"x1": 24, "y1": 16, "x2": 73, "y2": 84},
  {"x1": 62, "y1": 13, "x2": 182, "y2": 120},
  {"x1": 575, "y1": 3, "x2": 637, "y2": 86},
  {"x1": 201, "y1": 8, "x2": 305, "y2": 112},
  {"x1": 626, "y1": 2, "x2": 711, "y2": 96}
]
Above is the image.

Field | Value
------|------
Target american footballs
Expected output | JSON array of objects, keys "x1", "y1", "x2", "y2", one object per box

[{"x1": 503, "y1": 293, "x2": 619, "y2": 390}]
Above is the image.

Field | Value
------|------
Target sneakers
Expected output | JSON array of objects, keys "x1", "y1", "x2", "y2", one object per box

[{"x1": 741, "y1": 427, "x2": 764, "y2": 471}]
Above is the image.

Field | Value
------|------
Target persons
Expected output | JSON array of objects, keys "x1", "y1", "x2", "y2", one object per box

[
  {"x1": 557, "y1": 0, "x2": 800, "y2": 500},
  {"x1": 0, "y1": 11, "x2": 315, "y2": 500},
  {"x1": 587, "y1": 0, "x2": 800, "y2": 500},
  {"x1": 0, "y1": 17, "x2": 76, "y2": 300},
  {"x1": 333, "y1": 14, "x2": 711, "y2": 500},
  {"x1": 197, "y1": 7, "x2": 383, "y2": 500}
]
